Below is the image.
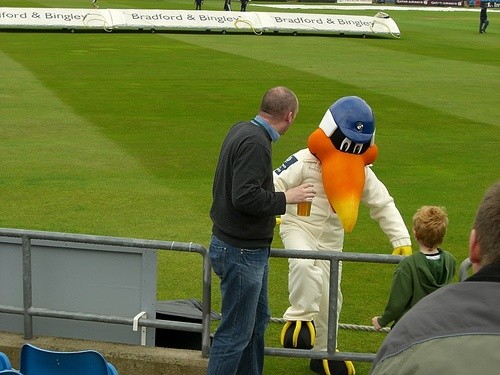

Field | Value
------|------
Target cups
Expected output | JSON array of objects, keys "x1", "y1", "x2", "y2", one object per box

[{"x1": 296, "y1": 198, "x2": 312, "y2": 217}]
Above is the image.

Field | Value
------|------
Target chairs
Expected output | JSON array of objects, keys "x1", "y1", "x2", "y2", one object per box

[{"x1": 19, "y1": 342, "x2": 112, "y2": 375}]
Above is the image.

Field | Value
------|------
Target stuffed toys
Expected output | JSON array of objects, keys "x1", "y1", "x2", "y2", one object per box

[{"x1": 272, "y1": 96, "x2": 413, "y2": 375}]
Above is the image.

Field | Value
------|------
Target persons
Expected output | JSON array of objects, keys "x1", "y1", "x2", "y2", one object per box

[
  {"x1": 479, "y1": 4, "x2": 489, "y2": 35},
  {"x1": 206, "y1": 85, "x2": 298, "y2": 375},
  {"x1": 195, "y1": 0, "x2": 249, "y2": 12},
  {"x1": 370, "y1": 183, "x2": 500, "y2": 375},
  {"x1": 372, "y1": 205, "x2": 457, "y2": 332}
]
